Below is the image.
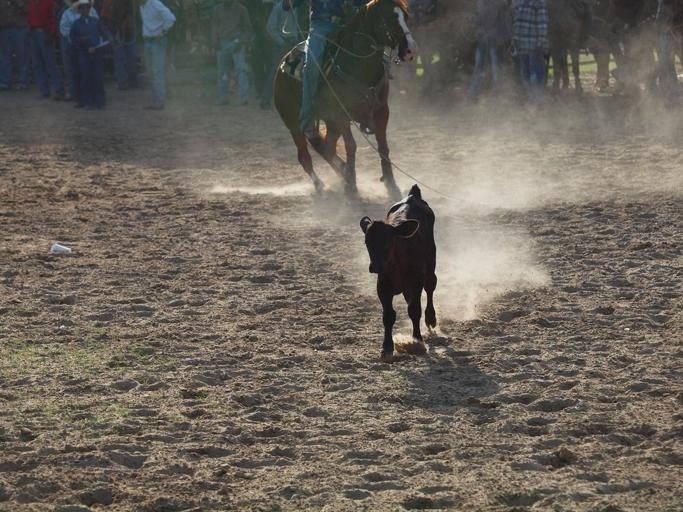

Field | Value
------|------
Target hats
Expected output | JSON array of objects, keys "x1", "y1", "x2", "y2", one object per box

[{"x1": 73, "y1": 0, "x2": 94, "y2": 11}]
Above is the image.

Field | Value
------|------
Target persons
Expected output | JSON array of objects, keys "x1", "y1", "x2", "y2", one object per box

[
  {"x1": 298, "y1": 0, "x2": 361, "y2": 133},
  {"x1": 395, "y1": 0, "x2": 683, "y2": 108},
  {"x1": 0, "y1": 0, "x2": 311, "y2": 114}
]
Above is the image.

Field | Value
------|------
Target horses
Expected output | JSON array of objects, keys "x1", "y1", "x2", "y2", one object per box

[
  {"x1": 397, "y1": 0, "x2": 683, "y2": 107},
  {"x1": 270, "y1": 0, "x2": 420, "y2": 201}
]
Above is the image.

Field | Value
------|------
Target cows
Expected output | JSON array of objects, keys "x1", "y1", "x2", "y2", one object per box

[{"x1": 357, "y1": 182, "x2": 439, "y2": 359}]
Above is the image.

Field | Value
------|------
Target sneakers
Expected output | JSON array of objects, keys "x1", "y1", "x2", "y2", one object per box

[
  {"x1": 144, "y1": 103, "x2": 164, "y2": 110},
  {"x1": 300, "y1": 119, "x2": 316, "y2": 133},
  {"x1": 214, "y1": 92, "x2": 272, "y2": 113},
  {"x1": 0, "y1": 83, "x2": 104, "y2": 111}
]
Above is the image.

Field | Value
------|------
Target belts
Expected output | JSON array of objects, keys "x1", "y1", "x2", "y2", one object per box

[
  {"x1": 143, "y1": 35, "x2": 153, "y2": 41},
  {"x1": 327, "y1": 15, "x2": 344, "y2": 24}
]
